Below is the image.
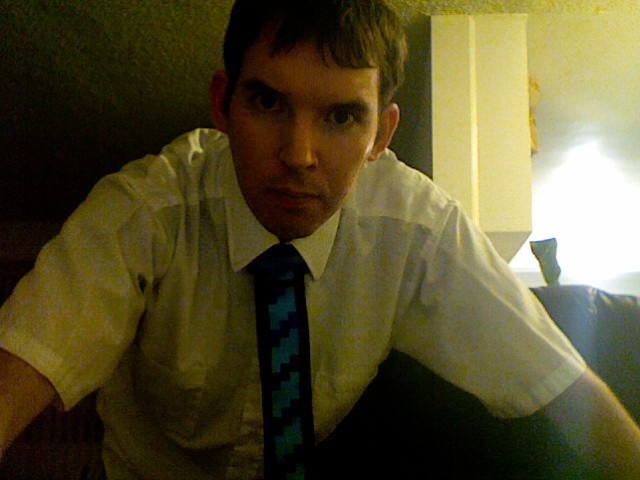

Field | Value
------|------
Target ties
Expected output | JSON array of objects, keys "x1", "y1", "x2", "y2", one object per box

[{"x1": 250, "y1": 243, "x2": 316, "y2": 479}]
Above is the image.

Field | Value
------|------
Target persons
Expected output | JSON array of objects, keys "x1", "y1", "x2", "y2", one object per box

[{"x1": 0, "y1": 0, "x2": 636, "y2": 478}]
